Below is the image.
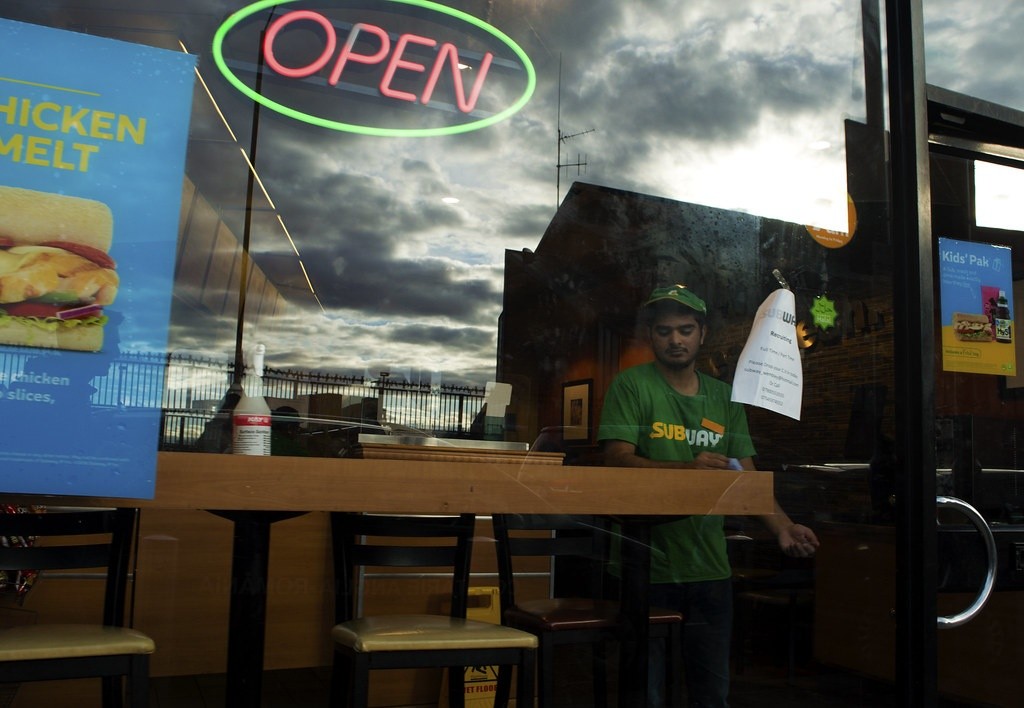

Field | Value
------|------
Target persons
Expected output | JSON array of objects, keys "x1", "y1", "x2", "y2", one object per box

[{"x1": 597, "y1": 284, "x2": 819, "y2": 708}]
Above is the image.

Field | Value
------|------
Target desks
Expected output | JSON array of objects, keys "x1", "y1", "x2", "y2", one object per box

[{"x1": 155, "y1": 409, "x2": 393, "y2": 708}]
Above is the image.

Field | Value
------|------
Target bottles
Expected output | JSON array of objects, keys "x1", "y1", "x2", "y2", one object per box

[
  {"x1": 231, "y1": 366, "x2": 272, "y2": 456},
  {"x1": 995, "y1": 291, "x2": 1011, "y2": 343}
]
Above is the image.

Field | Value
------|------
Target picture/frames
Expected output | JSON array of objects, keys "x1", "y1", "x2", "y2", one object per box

[{"x1": 559, "y1": 377, "x2": 593, "y2": 445}]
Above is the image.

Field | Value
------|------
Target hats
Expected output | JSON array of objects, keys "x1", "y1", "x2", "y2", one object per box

[{"x1": 645, "y1": 285, "x2": 707, "y2": 318}]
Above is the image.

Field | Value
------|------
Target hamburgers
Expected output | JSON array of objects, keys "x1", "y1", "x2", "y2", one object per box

[
  {"x1": 953, "y1": 312, "x2": 992, "y2": 342},
  {"x1": 0, "y1": 185, "x2": 120, "y2": 352}
]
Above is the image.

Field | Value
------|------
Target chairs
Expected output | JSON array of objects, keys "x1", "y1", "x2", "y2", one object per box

[
  {"x1": 492, "y1": 513, "x2": 685, "y2": 708},
  {"x1": 0, "y1": 505, "x2": 154, "y2": 708},
  {"x1": 327, "y1": 509, "x2": 539, "y2": 708}
]
